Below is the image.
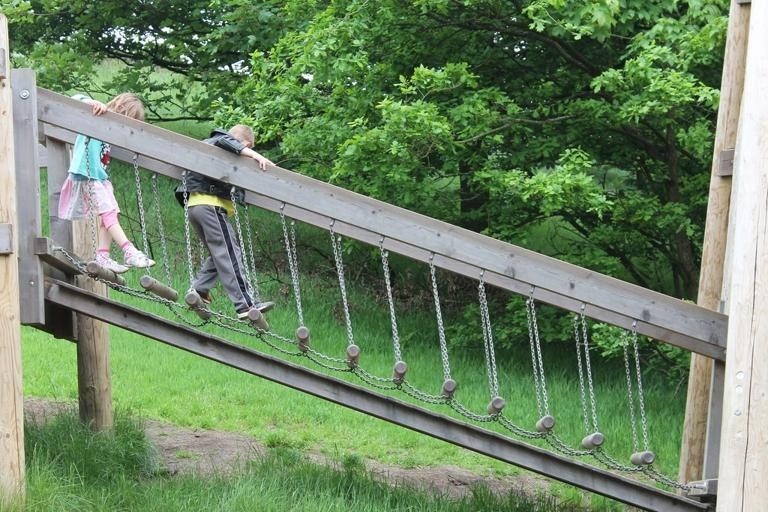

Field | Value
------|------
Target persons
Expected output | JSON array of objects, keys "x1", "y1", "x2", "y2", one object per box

[
  {"x1": 174, "y1": 122, "x2": 275, "y2": 326},
  {"x1": 55, "y1": 91, "x2": 160, "y2": 273}
]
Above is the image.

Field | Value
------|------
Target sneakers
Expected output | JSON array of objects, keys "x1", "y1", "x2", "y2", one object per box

[
  {"x1": 97, "y1": 250, "x2": 155, "y2": 273},
  {"x1": 196, "y1": 291, "x2": 212, "y2": 304},
  {"x1": 238, "y1": 301, "x2": 275, "y2": 320}
]
